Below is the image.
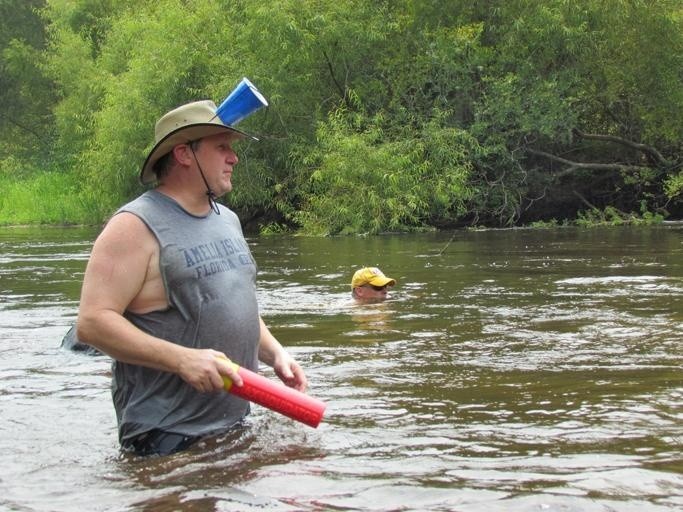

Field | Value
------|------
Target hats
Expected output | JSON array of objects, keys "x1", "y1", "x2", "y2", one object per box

[
  {"x1": 352, "y1": 267, "x2": 396, "y2": 288},
  {"x1": 139, "y1": 100, "x2": 260, "y2": 185}
]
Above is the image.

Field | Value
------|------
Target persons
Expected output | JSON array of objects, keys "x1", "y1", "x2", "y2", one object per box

[
  {"x1": 351, "y1": 266, "x2": 397, "y2": 300},
  {"x1": 75, "y1": 99, "x2": 308, "y2": 458}
]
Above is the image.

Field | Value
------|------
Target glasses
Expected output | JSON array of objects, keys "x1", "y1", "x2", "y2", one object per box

[{"x1": 365, "y1": 285, "x2": 384, "y2": 291}]
